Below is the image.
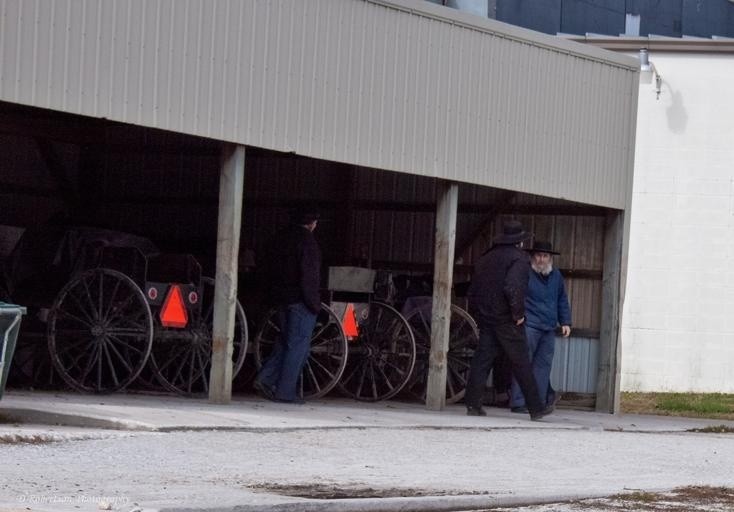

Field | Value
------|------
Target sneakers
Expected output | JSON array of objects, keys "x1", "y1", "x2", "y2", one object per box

[
  {"x1": 254, "y1": 380, "x2": 280, "y2": 401},
  {"x1": 467, "y1": 406, "x2": 487, "y2": 416},
  {"x1": 276, "y1": 397, "x2": 305, "y2": 404},
  {"x1": 511, "y1": 390, "x2": 563, "y2": 418}
]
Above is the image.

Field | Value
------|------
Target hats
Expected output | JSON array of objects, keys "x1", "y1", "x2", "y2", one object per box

[
  {"x1": 522, "y1": 242, "x2": 561, "y2": 255},
  {"x1": 492, "y1": 220, "x2": 535, "y2": 244}
]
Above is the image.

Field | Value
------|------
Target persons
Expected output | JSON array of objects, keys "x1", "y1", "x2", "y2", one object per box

[
  {"x1": 508, "y1": 240, "x2": 573, "y2": 415},
  {"x1": 252, "y1": 208, "x2": 323, "y2": 405},
  {"x1": 465, "y1": 220, "x2": 553, "y2": 422}
]
